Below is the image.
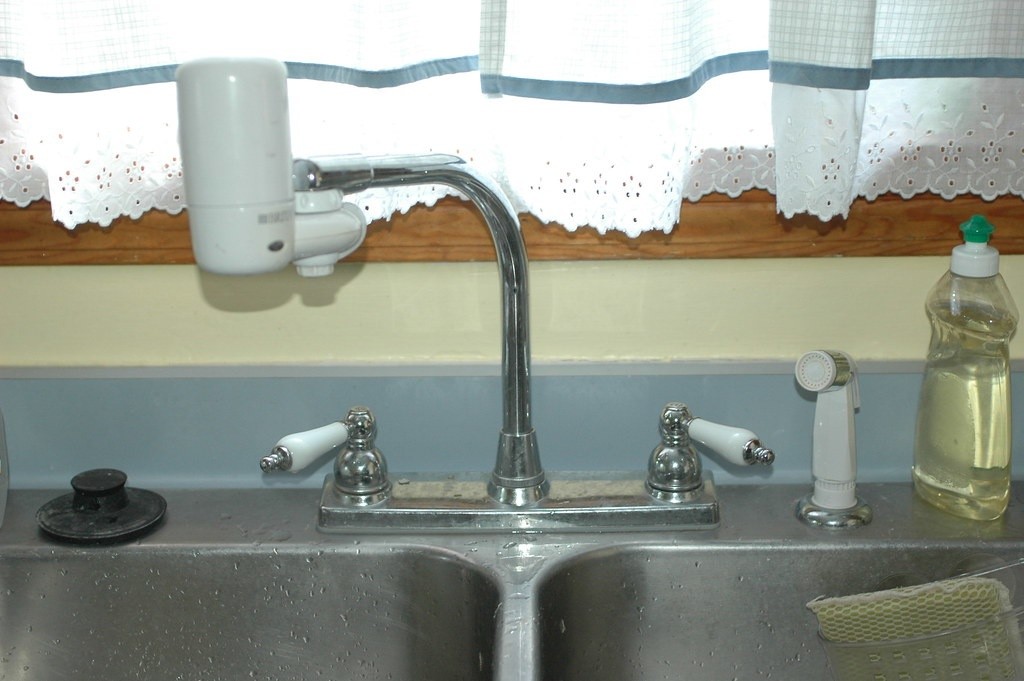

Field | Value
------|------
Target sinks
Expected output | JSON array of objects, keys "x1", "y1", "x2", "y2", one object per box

[
  {"x1": 529, "y1": 539, "x2": 1024, "y2": 681},
  {"x1": 1, "y1": 538, "x2": 507, "y2": 681}
]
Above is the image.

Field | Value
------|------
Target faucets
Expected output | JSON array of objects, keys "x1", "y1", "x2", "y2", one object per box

[{"x1": 173, "y1": 53, "x2": 557, "y2": 511}]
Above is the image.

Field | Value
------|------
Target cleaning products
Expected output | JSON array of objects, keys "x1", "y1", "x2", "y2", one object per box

[{"x1": 908, "y1": 208, "x2": 1019, "y2": 524}]
